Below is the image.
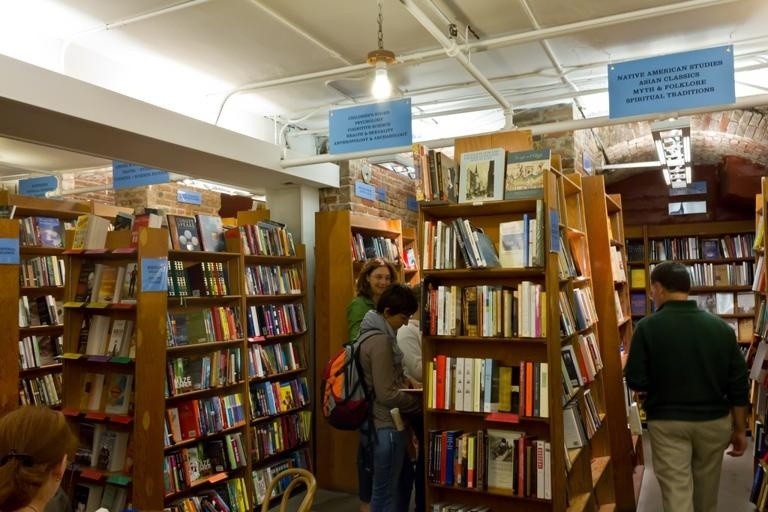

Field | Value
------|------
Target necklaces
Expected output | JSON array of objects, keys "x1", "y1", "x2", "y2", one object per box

[{"x1": 28, "y1": 504, "x2": 36, "y2": 511}]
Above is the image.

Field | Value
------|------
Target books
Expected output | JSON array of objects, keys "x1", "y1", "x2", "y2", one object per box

[
  {"x1": 1, "y1": 204, "x2": 73, "y2": 405},
  {"x1": 610, "y1": 244, "x2": 647, "y2": 436},
  {"x1": 74, "y1": 208, "x2": 314, "y2": 512},
  {"x1": 413, "y1": 145, "x2": 552, "y2": 499},
  {"x1": 558, "y1": 235, "x2": 605, "y2": 469},
  {"x1": 648, "y1": 236, "x2": 735, "y2": 315},
  {"x1": 716, "y1": 177, "x2": 768, "y2": 512},
  {"x1": 351, "y1": 234, "x2": 415, "y2": 269}
]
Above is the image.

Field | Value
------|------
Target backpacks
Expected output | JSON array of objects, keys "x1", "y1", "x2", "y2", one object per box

[{"x1": 320, "y1": 329, "x2": 385, "y2": 431}]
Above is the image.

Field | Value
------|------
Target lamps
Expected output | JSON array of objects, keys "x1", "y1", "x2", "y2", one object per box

[
  {"x1": 364, "y1": 1, "x2": 396, "y2": 105},
  {"x1": 600, "y1": 118, "x2": 694, "y2": 191}
]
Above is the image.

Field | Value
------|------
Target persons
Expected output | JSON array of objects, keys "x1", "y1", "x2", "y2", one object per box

[
  {"x1": 1, "y1": 408, "x2": 81, "y2": 511},
  {"x1": 346, "y1": 259, "x2": 423, "y2": 511},
  {"x1": 624, "y1": 263, "x2": 750, "y2": 511}
]
